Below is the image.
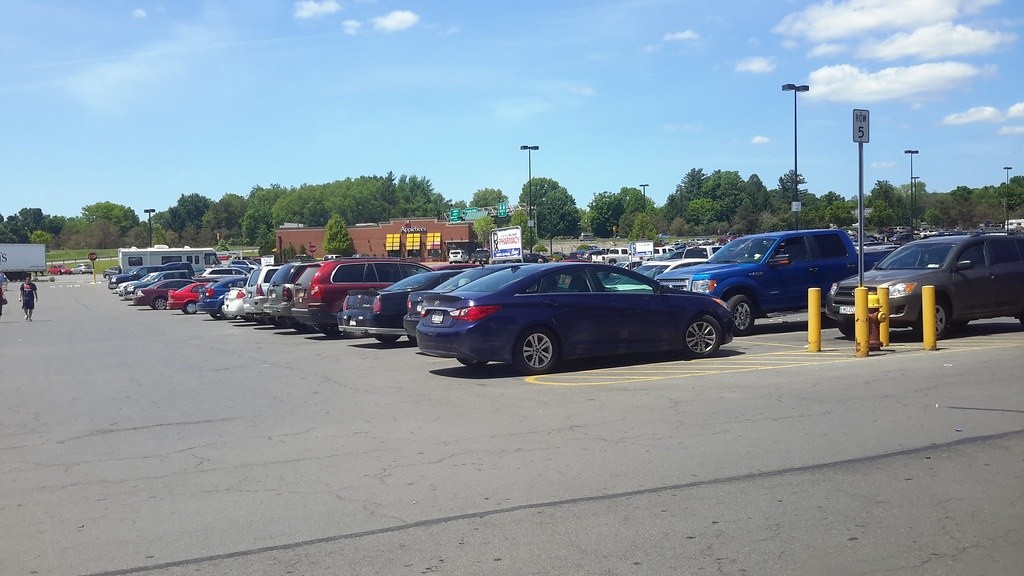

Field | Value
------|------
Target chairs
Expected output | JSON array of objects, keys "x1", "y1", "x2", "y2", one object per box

[
  {"x1": 539, "y1": 276, "x2": 561, "y2": 292},
  {"x1": 568, "y1": 276, "x2": 606, "y2": 293}
]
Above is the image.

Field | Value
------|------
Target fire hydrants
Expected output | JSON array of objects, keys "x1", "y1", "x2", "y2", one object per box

[{"x1": 859, "y1": 294, "x2": 888, "y2": 353}]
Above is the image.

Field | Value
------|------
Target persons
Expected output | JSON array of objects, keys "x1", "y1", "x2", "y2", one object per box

[
  {"x1": 0, "y1": 269, "x2": 7, "y2": 315},
  {"x1": 19, "y1": 277, "x2": 38, "y2": 321}
]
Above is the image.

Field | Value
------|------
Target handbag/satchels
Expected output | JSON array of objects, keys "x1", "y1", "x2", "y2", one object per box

[{"x1": 2, "y1": 299, "x2": 7, "y2": 305}]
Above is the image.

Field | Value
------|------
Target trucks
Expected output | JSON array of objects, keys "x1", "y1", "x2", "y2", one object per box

[{"x1": 1, "y1": 244, "x2": 46, "y2": 282}]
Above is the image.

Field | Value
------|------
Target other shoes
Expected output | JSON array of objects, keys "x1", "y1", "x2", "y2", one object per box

[
  {"x1": 28, "y1": 317, "x2": 32, "y2": 322},
  {"x1": 24, "y1": 315, "x2": 28, "y2": 321}
]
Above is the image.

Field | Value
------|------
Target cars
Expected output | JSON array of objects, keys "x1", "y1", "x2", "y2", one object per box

[
  {"x1": 521, "y1": 253, "x2": 549, "y2": 264},
  {"x1": 545, "y1": 250, "x2": 565, "y2": 262},
  {"x1": 71, "y1": 264, "x2": 93, "y2": 275},
  {"x1": 571, "y1": 244, "x2": 642, "y2": 272},
  {"x1": 338, "y1": 268, "x2": 466, "y2": 346},
  {"x1": 102, "y1": 261, "x2": 285, "y2": 327},
  {"x1": 47, "y1": 263, "x2": 71, "y2": 276},
  {"x1": 630, "y1": 257, "x2": 708, "y2": 283},
  {"x1": 579, "y1": 232, "x2": 595, "y2": 241},
  {"x1": 403, "y1": 261, "x2": 613, "y2": 352},
  {"x1": 415, "y1": 262, "x2": 736, "y2": 376},
  {"x1": 654, "y1": 237, "x2": 725, "y2": 261}
]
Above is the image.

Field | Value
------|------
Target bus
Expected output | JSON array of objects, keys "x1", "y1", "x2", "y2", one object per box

[{"x1": 119, "y1": 247, "x2": 223, "y2": 280}]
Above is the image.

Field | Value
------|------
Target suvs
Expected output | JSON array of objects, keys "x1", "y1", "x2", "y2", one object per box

[
  {"x1": 824, "y1": 233, "x2": 1024, "y2": 341},
  {"x1": 291, "y1": 258, "x2": 435, "y2": 341},
  {"x1": 473, "y1": 248, "x2": 490, "y2": 264},
  {"x1": 655, "y1": 228, "x2": 859, "y2": 338},
  {"x1": 261, "y1": 260, "x2": 326, "y2": 333}
]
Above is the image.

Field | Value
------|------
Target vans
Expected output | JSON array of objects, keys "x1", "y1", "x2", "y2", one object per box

[{"x1": 449, "y1": 249, "x2": 468, "y2": 263}]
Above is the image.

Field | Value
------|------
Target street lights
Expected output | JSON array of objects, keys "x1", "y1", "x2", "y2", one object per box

[
  {"x1": 144, "y1": 208, "x2": 155, "y2": 247},
  {"x1": 520, "y1": 145, "x2": 539, "y2": 253},
  {"x1": 905, "y1": 149, "x2": 919, "y2": 241},
  {"x1": 640, "y1": 184, "x2": 649, "y2": 214},
  {"x1": 782, "y1": 83, "x2": 810, "y2": 228},
  {"x1": 1003, "y1": 166, "x2": 1013, "y2": 234}
]
